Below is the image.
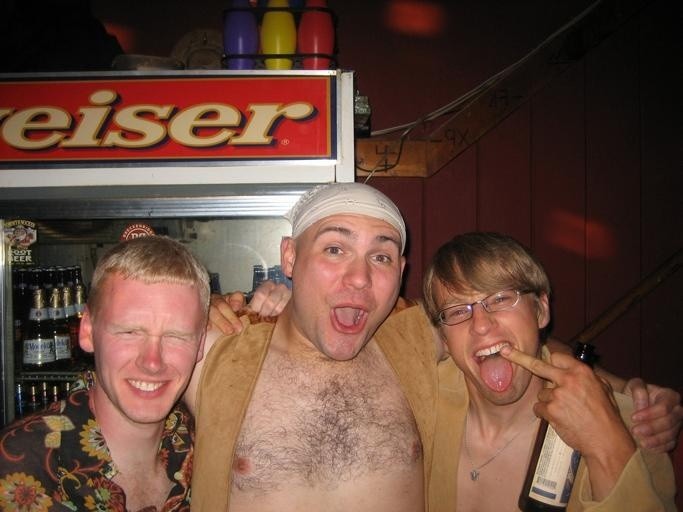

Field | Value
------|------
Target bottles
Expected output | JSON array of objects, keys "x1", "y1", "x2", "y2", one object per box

[
  {"x1": 517, "y1": 339, "x2": 599, "y2": 511},
  {"x1": 16, "y1": 266, "x2": 87, "y2": 416},
  {"x1": 209, "y1": 263, "x2": 292, "y2": 305}
]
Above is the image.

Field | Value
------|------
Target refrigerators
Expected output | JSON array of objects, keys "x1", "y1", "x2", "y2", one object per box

[{"x1": 1, "y1": 66, "x2": 356, "y2": 428}]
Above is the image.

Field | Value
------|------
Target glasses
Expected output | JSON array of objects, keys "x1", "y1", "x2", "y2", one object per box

[{"x1": 430, "y1": 288, "x2": 536, "y2": 327}]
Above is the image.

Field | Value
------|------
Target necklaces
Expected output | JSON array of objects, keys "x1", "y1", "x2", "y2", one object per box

[{"x1": 464, "y1": 408, "x2": 535, "y2": 480}]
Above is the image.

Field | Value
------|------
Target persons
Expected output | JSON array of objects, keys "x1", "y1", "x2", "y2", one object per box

[
  {"x1": 179, "y1": 182, "x2": 682, "y2": 512},
  {"x1": 0, "y1": 235, "x2": 290, "y2": 512},
  {"x1": 205, "y1": 228, "x2": 679, "y2": 512}
]
